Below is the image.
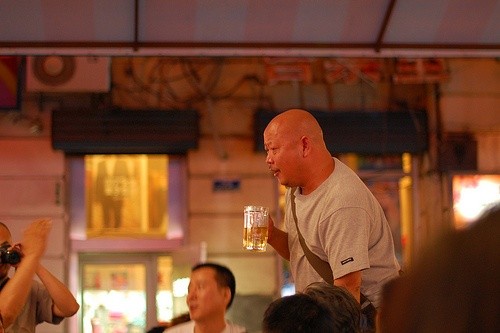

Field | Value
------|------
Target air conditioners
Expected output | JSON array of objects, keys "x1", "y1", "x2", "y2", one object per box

[{"x1": 26, "y1": 57, "x2": 110, "y2": 92}]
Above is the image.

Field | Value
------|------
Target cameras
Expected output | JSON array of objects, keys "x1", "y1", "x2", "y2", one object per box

[{"x1": 0, "y1": 240, "x2": 20, "y2": 265}]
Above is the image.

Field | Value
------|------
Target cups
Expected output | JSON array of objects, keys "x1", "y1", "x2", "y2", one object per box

[
  {"x1": 108, "y1": 313, "x2": 124, "y2": 333},
  {"x1": 242, "y1": 206, "x2": 269, "y2": 254},
  {"x1": 91, "y1": 319, "x2": 101, "y2": 333}
]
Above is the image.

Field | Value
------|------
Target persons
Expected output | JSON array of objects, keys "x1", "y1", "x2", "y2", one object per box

[
  {"x1": 148, "y1": 262, "x2": 247, "y2": 333},
  {"x1": 0, "y1": 217, "x2": 80, "y2": 333},
  {"x1": 262, "y1": 202, "x2": 500, "y2": 333},
  {"x1": 257, "y1": 109, "x2": 402, "y2": 333}
]
